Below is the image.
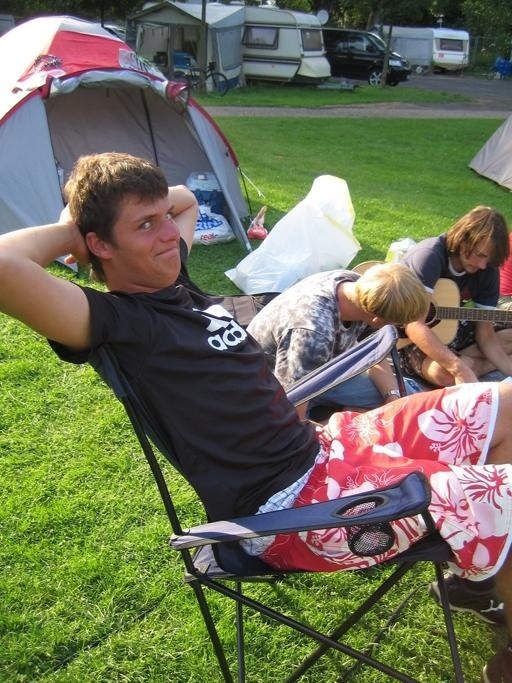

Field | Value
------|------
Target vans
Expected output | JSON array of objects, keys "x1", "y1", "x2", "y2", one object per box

[{"x1": 322, "y1": 27, "x2": 412, "y2": 88}]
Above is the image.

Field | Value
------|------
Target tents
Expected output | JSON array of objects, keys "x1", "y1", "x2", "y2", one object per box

[
  {"x1": 466, "y1": 111, "x2": 511, "y2": 192},
  {"x1": 1, "y1": 14, "x2": 255, "y2": 271}
]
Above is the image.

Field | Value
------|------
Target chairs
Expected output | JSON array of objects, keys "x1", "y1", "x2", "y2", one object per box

[{"x1": 97, "y1": 324, "x2": 463, "y2": 683}]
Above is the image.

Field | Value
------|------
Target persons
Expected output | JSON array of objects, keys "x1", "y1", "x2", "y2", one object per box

[
  {"x1": 495, "y1": 231, "x2": 512, "y2": 311},
  {"x1": 241, "y1": 260, "x2": 433, "y2": 424},
  {"x1": 0, "y1": 151, "x2": 512, "y2": 683},
  {"x1": 394, "y1": 203, "x2": 512, "y2": 387}
]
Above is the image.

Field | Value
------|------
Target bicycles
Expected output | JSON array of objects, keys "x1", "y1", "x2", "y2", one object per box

[{"x1": 174, "y1": 56, "x2": 229, "y2": 97}]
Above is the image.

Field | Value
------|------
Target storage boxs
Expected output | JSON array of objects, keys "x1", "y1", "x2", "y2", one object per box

[{"x1": 185, "y1": 170, "x2": 224, "y2": 213}]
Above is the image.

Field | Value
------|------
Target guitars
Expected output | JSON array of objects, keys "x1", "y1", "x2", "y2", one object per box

[{"x1": 352, "y1": 260, "x2": 512, "y2": 352}]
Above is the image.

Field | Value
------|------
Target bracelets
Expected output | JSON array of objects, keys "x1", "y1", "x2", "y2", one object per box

[{"x1": 382, "y1": 388, "x2": 400, "y2": 403}]
